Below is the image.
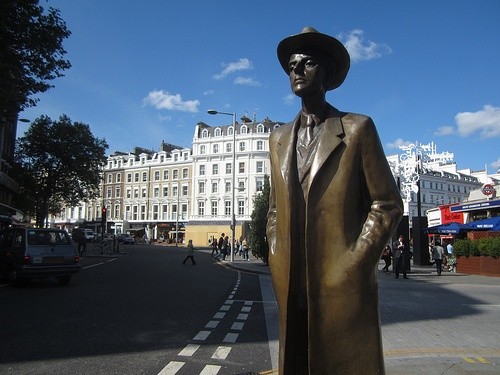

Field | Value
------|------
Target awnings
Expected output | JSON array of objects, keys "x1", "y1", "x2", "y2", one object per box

[{"x1": 127, "y1": 227, "x2": 145, "y2": 232}]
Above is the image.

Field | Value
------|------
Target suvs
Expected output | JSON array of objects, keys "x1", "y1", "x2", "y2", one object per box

[{"x1": 117, "y1": 233, "x2": 135, "y2": 245}]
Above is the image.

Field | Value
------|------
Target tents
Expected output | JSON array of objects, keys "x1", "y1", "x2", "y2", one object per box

[{"x1": 424, "y1": 216, "x2": 498, "y2": 234}]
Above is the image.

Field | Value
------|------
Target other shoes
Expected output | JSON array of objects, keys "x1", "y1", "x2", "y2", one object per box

[
  {"x1": 404, "y1": 276, "x2": 408, "y2": 279},
  {"x1": 394, "y1": 276, "x2": 398, "y2": 278},
  {"x1": 382, "y1": 268, "x2": 385, "y2": 273},
  {"x1": 387, "y1": 271, "x2": 390, "y2": 273}
]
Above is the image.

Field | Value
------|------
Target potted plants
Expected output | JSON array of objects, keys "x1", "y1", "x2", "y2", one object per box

[
  {"x1": 454, "y1": 238, "x2": 480, "y2": 273},
  {"x1": 479, "y1": 233, "x2": 500, "y2": 273}
]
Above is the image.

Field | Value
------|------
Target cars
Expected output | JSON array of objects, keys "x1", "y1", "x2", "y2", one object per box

[{"x1": 0, "y1": 227, "x2": 80, "y2": 287}]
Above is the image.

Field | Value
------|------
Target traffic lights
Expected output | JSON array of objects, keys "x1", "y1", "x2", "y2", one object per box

[{"x1": 101, "y1": 208, "x2": 106, "y2": 224}]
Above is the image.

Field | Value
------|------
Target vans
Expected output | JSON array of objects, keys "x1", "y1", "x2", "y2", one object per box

[{"x1": 82, "y1": 229, "x2": 97, "y2": 242}]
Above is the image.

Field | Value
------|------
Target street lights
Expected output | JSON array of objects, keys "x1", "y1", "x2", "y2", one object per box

[{"x1": 206, "y1": 109, "x2": 237, "y2": 263}]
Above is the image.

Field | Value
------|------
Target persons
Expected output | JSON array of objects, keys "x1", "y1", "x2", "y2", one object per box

[
  {"x1": 381, "y1": 234, "x2": 453, "y2": 279},
  {"x1": 182, "y1": 240, "x2": 196, "y2": 265},
  {"x1": 78, "y1": 232, "x2": 87, "y2": 257},
  {"x1": 266, "y1": 26, "x2": 404, "y2": 375},
  {"x1": 209, "y1": 233, "x2": 250, "y2": 261}
]
